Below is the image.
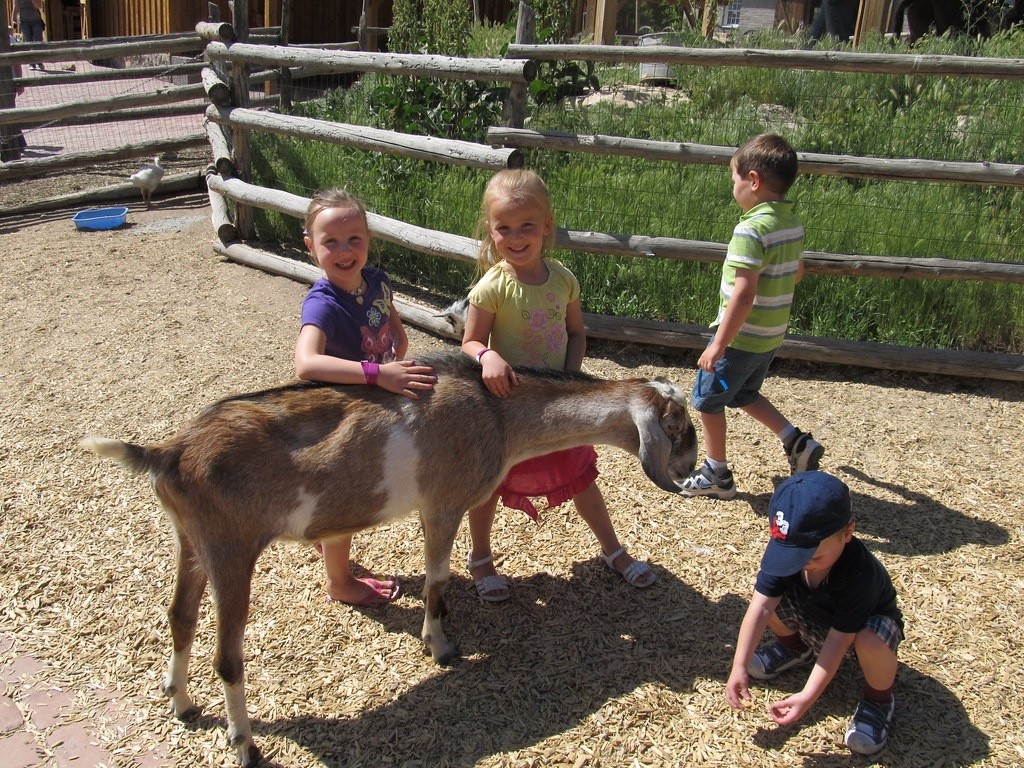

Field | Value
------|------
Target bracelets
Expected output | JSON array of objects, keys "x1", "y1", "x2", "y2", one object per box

[
  {"x1": 476, "y1": 348, "x2": 490, "y2": 362},
  {"x1": 361, "y1": 360, "x2": 379, "y2": 384}
]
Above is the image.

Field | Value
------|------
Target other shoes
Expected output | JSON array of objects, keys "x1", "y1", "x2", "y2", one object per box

[
  {"x1": 39, "y1": 63, "x2": 44, "y2": 68},
  {"x1": 29, "y1": 65, "x2": 35, "y2": 70}
]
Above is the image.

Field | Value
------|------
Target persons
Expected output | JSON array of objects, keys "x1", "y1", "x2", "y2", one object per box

[
  {"x1": 725, "y1": 471, "x2": 905, "y2": 756},
  {"x1": 460, "y1": 169, "x2": 658, "y2": 602},
  {"x1": 677, "y1": 132, "x2": 826, "y2": 501},
  {"x1": 8, "y1": 25, "x2": 27, "y2": 153},
  {"x1": 296, "y1": 190, "x2": 438, "y2": 608},
  {"x1": 11, "y1": 0, "x2": 46, "y2": 71}
]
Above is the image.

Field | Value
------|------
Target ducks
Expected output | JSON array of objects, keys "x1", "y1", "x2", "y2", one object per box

[{"x1": 130, "y1": 155, "x2": 165, "y2": 212}]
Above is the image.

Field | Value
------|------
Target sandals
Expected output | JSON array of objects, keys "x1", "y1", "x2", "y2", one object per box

[
  {"x1": 601, "y1": 544, "x2": 656, "y2": 588},
  {"x1": 468, "y1": 549, "x2": 510, "y2": 601}
]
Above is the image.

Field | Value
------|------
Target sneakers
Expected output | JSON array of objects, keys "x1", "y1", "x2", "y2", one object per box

[
  {"x1": 845, "y1": 693, "x2": 895, "y2": 755},
  {"x1": 746, "y1": 642, "x2": 814, "y2": 679},
  {"x1": 673, "y1": 465, "x2": 737, "y2": 500},
  {"x1": 782, "y1": 426, "x2": 824, "y2": 476}
]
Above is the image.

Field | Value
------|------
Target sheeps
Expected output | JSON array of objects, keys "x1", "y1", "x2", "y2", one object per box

[{"x1": 71, "y1": 349, "x2": 699, "y2": 768}]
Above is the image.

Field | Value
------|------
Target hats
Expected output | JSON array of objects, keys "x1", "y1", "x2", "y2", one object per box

[{"x1": 760, "y1": 471, "x2": 851, "y2": 577}]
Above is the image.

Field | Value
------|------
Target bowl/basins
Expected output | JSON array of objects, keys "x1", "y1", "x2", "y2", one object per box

[{"x1": 72, "y1": 207, "x2": 128, "y2": 230}]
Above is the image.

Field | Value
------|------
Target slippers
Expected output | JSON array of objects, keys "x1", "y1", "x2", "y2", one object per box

[
  {"x1": 327, "y1": 578, "x2": 401, "y2": 606},
  {"x1": 313, "y1": 542, "x2": 324, "y2": 555}
]
